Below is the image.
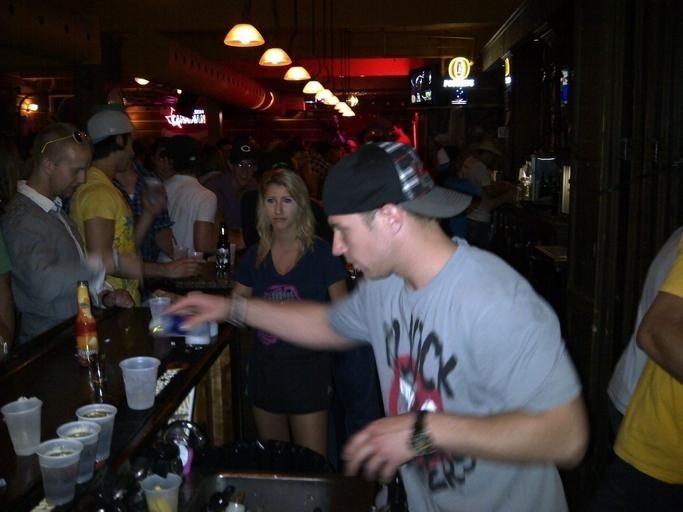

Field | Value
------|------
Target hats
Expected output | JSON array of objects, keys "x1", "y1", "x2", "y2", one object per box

[
  {"x1": 325, "y1": 141, "x2": 472, "y2": 218},
  {"x1": 229, "y1": 141, "x2": 255, "y2": 163}
]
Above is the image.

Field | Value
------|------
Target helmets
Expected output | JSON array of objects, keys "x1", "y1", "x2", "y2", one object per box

[{"x1": 86, "y1": 110, "x2": 132, "y2": 144}]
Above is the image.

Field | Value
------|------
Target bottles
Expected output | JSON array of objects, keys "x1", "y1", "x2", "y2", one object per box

[
  {"x1": 70, "y1": 278, "x2": 99, "y2": 367},
  {"x1": 147, "y1": 314, "x2": 211, "y2": 338},
  {"x1": 214, "y1": 223, "x2": 230, "y2": 271},
  {"x1": 490, "y1": 211, "x2": 511, "y2": 241},
  {"x1": 209, "y1": 487, "x2": 247, "y2": 511},
  {"x1": 184, "y1": 290, "x2": 220, "y2": 350}
]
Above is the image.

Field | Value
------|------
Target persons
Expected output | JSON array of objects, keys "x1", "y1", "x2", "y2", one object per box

[
  {"x1": 434, "y1": 131, "x2": 517, "y2": 246},
  {"x1": 161, "y1": 141, "x2": 589, "y2": 511},
  {"x1": 2, "y1": 109, "x2": 260, "y2": 356},
  {"x1": 591, "y1": 230, "x2": 681, "y2": 511},
  {"x1": 232, "y1": 135, "x2": 403, "y2": 510}
]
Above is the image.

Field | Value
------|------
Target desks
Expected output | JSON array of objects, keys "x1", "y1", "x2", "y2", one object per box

[{"x1": 1, "y1": 305, "x2": 251, "y2": 512}]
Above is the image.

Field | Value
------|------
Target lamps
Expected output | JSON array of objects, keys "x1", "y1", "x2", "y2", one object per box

[{"x1": 224, "y1": 0, "x2": 358, "y2": 117}]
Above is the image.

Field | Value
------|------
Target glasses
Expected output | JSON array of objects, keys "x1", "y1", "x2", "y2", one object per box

[{"x1": 41, "y1": 130, "x2": 91, "y2": 153}]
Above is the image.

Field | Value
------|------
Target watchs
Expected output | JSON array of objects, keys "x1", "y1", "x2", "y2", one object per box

[{"x1": 411, "y1": 409, "x2": 436, "y2": 456}]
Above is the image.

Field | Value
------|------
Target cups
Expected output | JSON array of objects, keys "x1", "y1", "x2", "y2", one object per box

[
  {"x1": 149, "y1": 296, "x2": 168, "y2": 320},
  {"x1": 229, "y1": 244, "x2": 236, "y2": 265},
  {"x1": 85, "y1": 218, "x2": 114, "y2": 255},
  {"x1": 186, "y1": 252, "x2": 203, "y2": 278},
  {"x1": 144, "y1": 177, "x2": 160, "y2": 205},
  {"x1": 141, "y1": 471, "x2": 183, "y2": 511},
  {"x1": 1, "y1": 397, "x2": 41, "y2": 458},
  {"x1": 36, "y1": 401, "x2": 115, "y2": 506},
  {"x1": 117, "y1": 355, "x2": 161, "y2": 410}
]
